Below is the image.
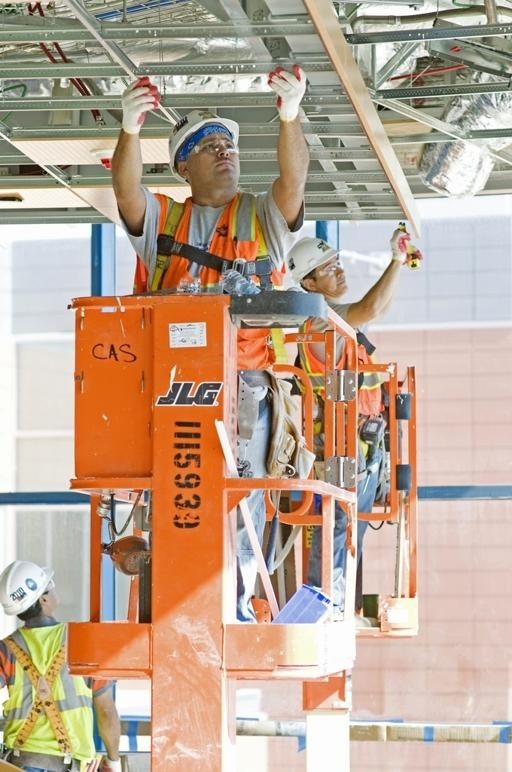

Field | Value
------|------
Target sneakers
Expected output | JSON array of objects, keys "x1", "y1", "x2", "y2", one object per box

[{"x1": 332, "y1": 613, "x2": 379, "y2": 627}]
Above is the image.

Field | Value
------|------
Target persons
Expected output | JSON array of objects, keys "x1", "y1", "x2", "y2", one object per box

[
  {"x1": 2, "y1": 559, "x2": 125, "y2": 772},
  {"x1": 288, "y1": 227, "x2": 414, "y2": 620},
  {"x1": 110, "y1": 64, "x2": 312, "y2": 623}
]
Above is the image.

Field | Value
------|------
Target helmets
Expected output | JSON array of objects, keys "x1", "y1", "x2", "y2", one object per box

[
  {"x1": 0, "y1": 559, "x2": 56, "y2": 616},
  {"x1": 167, "y1": 110, "x2": 240, "y2": 183},
  {"x1": 284, "y1": 236, "x2": 342, "y2": 284}
]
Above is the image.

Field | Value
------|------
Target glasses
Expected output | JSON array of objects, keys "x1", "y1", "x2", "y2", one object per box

[
  {"x1": 299, "y1": 258, "x2": 341, "y2": 281},
  {"x1": 186, "y1": 139, "x2": 240, "y2": 158}
]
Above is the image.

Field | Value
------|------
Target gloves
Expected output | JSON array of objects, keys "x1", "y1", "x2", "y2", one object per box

[
  {"x1": 121, "y1": 76, "x2": 161, "y2": 135},
  {"x1": 389, "y1": 230, "x2": 411, "y2": 262},
  {"x1": 267, "y1": 64, "x2": 307, "y2": 122}
]
